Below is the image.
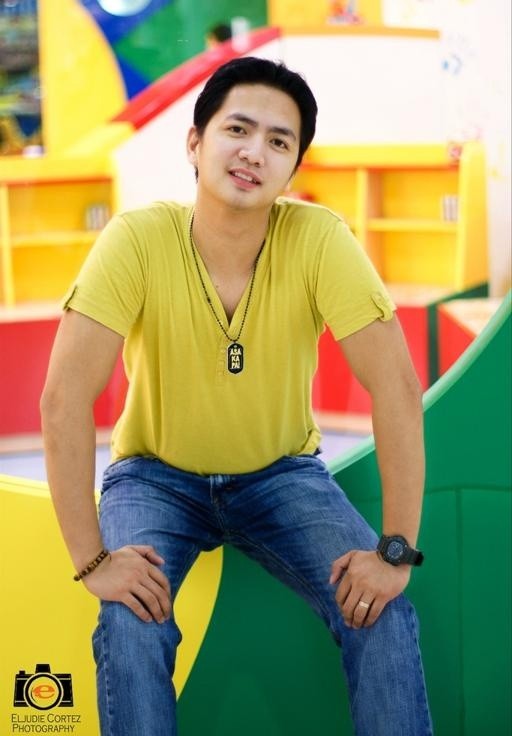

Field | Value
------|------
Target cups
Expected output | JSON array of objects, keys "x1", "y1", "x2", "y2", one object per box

[
  {"x1": 82, "y1": 201, "x2": 109, "y2": 232},
  {"x1": 442, "y1": 195, "x2": 458, "y2": 221}
]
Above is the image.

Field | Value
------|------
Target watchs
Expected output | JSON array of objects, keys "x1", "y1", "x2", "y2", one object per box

[{"x1": 376, "y1": 535, "x2": 424, "y2": 566}]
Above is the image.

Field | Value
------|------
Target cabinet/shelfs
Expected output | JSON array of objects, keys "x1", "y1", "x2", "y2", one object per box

[
  {"x1": 290, "y1": 140, "x2": 489, "y2": 298},
  {"x1": 0, "y1": 154, "x2": 122, "y2": 311}
]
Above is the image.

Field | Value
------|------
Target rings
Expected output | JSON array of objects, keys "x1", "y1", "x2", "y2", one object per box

[{"x1": 359, "y1": 601, "x2": 369, "y2": 609}]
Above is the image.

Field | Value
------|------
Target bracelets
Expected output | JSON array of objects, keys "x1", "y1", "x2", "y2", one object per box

[{"x1": 73, "y1": 547, "x2": 109, "y2": 582}]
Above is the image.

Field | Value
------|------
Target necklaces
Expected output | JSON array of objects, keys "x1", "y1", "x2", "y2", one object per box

[{"x1": 189, "y1": 215, "x2": 266, "y2": 374}]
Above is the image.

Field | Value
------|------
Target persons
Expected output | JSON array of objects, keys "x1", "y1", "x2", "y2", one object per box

[{"x1": 39, "y1": 57, "x2": 434, "y2": 735}]
